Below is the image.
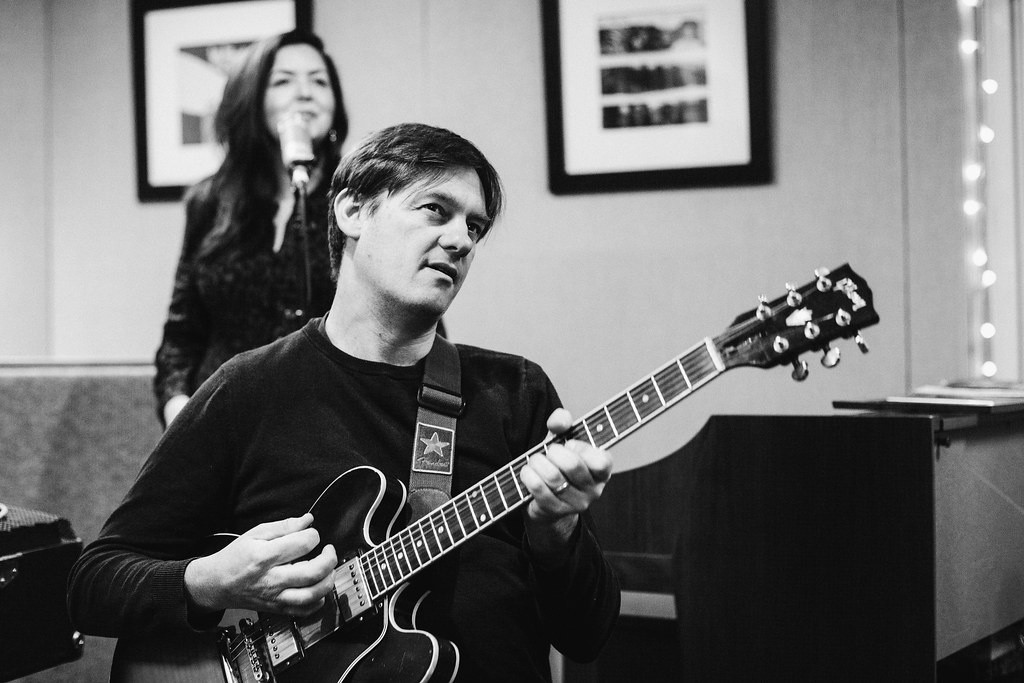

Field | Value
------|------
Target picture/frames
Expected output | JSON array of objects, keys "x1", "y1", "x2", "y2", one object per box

[
  {"x1": 539, "y1": 0, "x2": 775, "y2": 198},
  {"x1": 131, "y1": 0, "x2": 312, "y2": 198}
]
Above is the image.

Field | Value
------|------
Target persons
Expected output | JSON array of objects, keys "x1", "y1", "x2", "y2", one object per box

[
  {"x1": 154, "y1": 30, "x2": 349, "y2": 431},
  {"x1": 67, "y1": 124, "x2": 620, "y2": 683}
]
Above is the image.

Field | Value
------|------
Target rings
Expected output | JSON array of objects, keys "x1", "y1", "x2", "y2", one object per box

[{"x1": 555, "y1": 482, "x2": 568, "y2": 494}]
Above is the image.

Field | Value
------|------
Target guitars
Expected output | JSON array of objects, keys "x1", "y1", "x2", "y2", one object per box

[{"x1": 107, "y1": 263, "x2": 880, "y2": 683}]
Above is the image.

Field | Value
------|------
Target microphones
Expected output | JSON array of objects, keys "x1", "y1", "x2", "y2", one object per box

[{"x1": 274, "y1": 113, "x2": 317, "y2": 188}]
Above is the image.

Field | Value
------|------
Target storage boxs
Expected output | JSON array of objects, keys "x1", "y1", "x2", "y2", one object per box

[{"x1": 0, "y1": 506, "x2": 83, "y2": 683}]
